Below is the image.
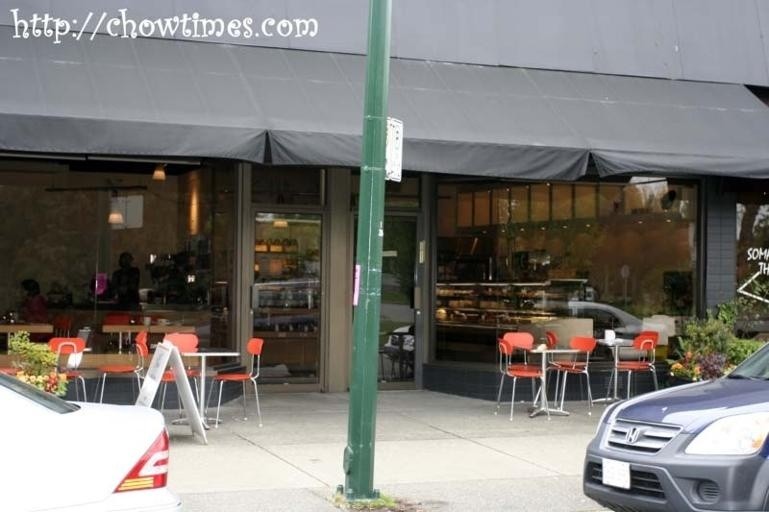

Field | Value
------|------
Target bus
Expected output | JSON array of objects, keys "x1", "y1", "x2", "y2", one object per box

[{"x1": 253, "y1": 279, "x2": 320, "y2": 334}]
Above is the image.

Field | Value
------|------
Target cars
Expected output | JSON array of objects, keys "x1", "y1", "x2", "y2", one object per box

[
  {"x1": 539, "y1": 302, "x2": 669, "y2": 362},
  {"x1": 578, "y1": 342, "x2": 769, "y2": 512},
  {"x1": 1, "y1": 370, "x2": 183, "y2": 505}
]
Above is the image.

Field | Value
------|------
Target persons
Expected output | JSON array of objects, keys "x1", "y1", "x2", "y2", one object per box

[
  {"x1": 20, "y1": 279, "x2": 49, "y2": 342},
  {"x1": 111, "y1": 253, "x2": 140, "y2": 311}
]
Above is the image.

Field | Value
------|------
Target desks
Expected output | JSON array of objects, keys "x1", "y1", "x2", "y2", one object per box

[{"x1": 381, "y1": 332, "x2": 415, "y2": 382}]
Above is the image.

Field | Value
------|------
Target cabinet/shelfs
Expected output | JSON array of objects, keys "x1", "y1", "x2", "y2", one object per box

[
  {"x1": 255, "y1": 238, "x2": 298, "y2": 282},
  {"x1": 140, "y1": 234, "x2": 216, "y2": 348},
  {"x1": 436, "y1": 279, "x2": 588, "y2": 325},
  {"x1": 455, "y1": 182, "x2": 698, "y2": 234}
]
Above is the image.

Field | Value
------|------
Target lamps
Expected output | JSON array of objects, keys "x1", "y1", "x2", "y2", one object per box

[
  {"x1": 108, "y1": 190, "x2": 123, "y2": 224},
  {"x1": 151, "y1": 163, "x2": 166, "y2": 180}
]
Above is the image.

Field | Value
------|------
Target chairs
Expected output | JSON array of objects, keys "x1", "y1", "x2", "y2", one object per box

[
  {"x1": 494, "y1": 330, "x2": 659, "y2": 421},
  {"x1": 0, "y1": 312, "x2": 265, "y2": 428},
  {"x1": 378, "y1": 347, "x2": 399, "y2": 382}
]
